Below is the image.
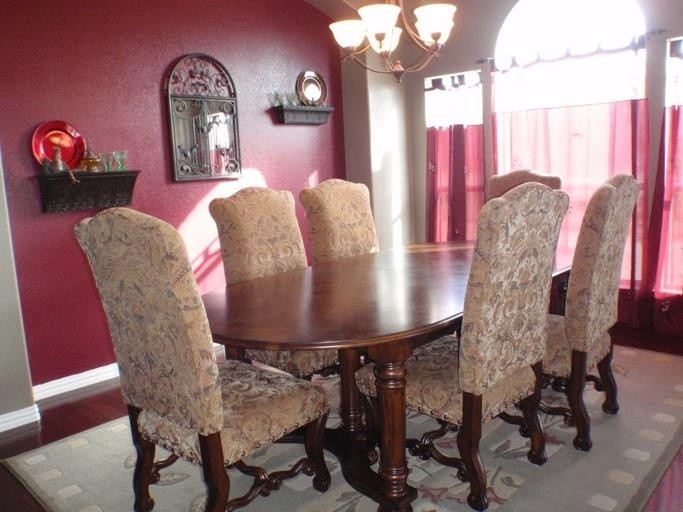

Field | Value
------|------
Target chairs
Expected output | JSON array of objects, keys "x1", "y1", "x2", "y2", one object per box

[
  {"x1": 299, "y1": 176, "x2": 379, "y2": 269},
  {"x1": 210, "y1": 184, "x2": 380, "y2": 465},
  {"x1": 513, "y1": 173, "x2": 641, "y2": 444},
  {"x1": 358, "y1": 177, "x2": 568, "y2": 511},
  {"x1": 483, "y1": 168, "x2": 563, "y2": 206},
  {"x1": 80, "y1": 206, "x2": 331, "y2": 510}
]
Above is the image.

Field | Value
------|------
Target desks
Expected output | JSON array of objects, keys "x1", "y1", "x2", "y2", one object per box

[{"x1": 200, "y1": 239, "x2": 587, "y2": 510}]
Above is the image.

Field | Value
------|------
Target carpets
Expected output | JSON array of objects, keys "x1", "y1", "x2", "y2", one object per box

[{"x1": 2, "y1": 342, "x2": 681, "y2": 511}]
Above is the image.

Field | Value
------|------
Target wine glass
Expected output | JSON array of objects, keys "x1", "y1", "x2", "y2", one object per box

[{"x1": 98, "y1": 149, "x2": 128, "y2": 172}]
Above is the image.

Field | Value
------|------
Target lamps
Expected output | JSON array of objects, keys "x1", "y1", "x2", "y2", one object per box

[{"x1": 327, "y1": 2, "x2": 459, "y2": 85}]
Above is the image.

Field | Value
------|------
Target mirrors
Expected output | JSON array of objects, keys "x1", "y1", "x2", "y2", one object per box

[{"x1": 169, "y1": 52, "x2": 241, "y2": 181}]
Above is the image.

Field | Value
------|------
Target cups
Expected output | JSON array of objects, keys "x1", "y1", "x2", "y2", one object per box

[{"x1": 272, "y1": 90, "x2": 301, "y2": 106}]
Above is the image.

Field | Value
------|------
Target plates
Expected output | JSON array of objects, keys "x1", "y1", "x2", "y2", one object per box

[
  {"x1": 30, "y1": 120, "x2": 85, "y2": 170},
  {"x1": 296, "y1": 70, "x2": 327, "y2": 107}
]
